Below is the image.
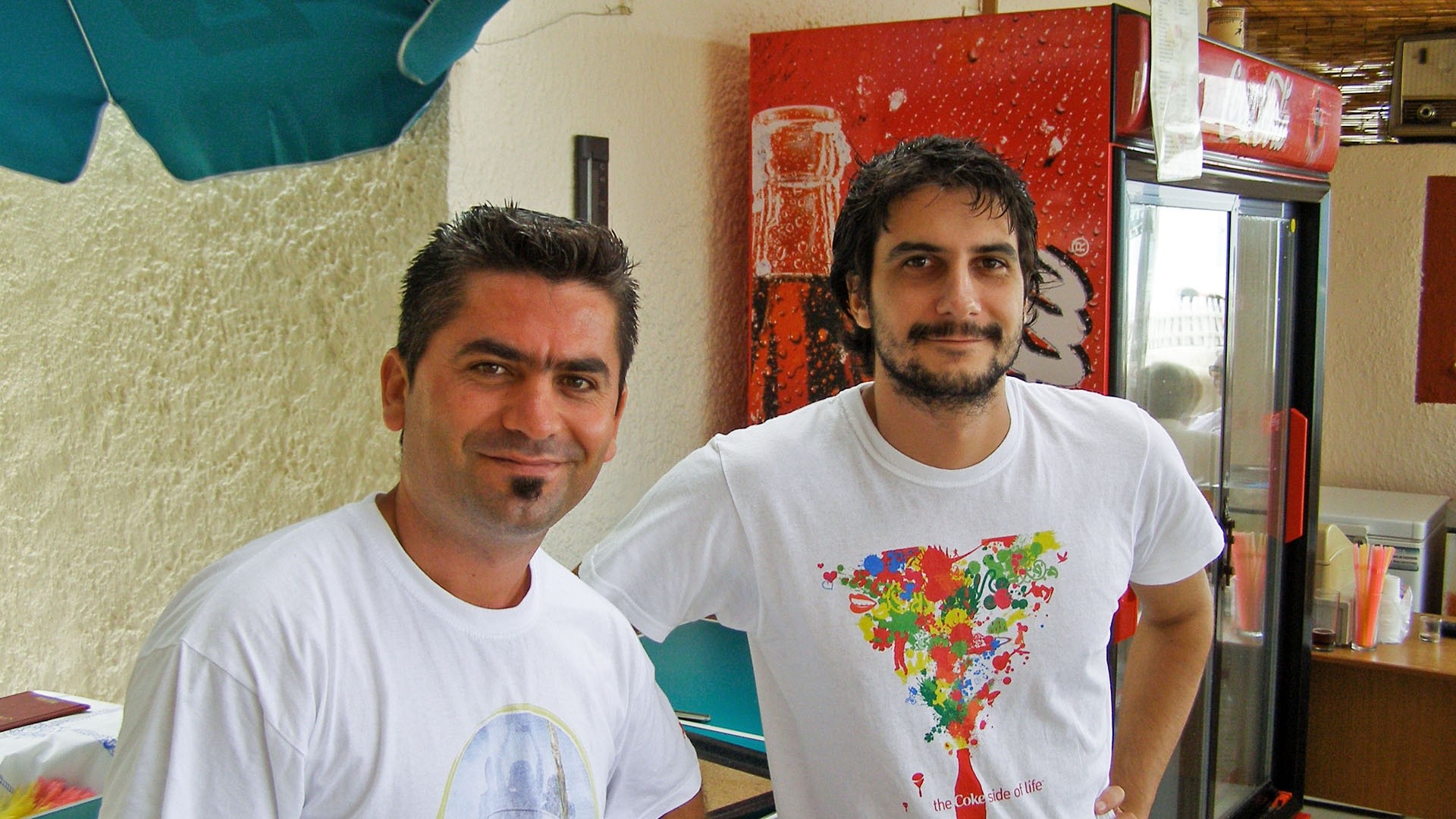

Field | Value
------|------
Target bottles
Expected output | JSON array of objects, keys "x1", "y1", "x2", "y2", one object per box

[{"x1": 747, "y1": 103, "x2": 875, "y2": 430}]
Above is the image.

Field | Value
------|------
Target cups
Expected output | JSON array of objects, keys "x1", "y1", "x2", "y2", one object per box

[
  {"x1": 1419, "y1": 615, "x2": 1442, "y2": 643},
  {"x1": 1312, "y1": 586, "x2": 1340, "y2": 652},
  {"x1": 1351, "y1": 590, "x2": 1382, "y2": 652}
]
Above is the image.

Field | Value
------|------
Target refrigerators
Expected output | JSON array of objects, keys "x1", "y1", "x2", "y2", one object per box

[{"x1": 747, "y1": 5, "x2": 1340, "y2": 819}]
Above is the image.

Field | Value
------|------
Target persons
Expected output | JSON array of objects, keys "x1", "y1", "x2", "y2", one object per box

[
  {"x1": 571, "y1": 137, "x2": 1225, "y2": 818},
  {"x1": 99, "y1": 204, "x2": 705, "y2": 819}
]
